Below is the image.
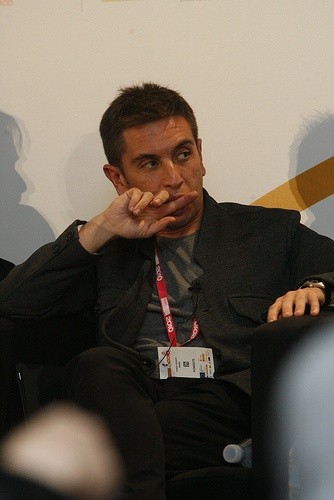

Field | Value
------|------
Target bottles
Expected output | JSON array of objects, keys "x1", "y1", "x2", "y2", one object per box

[{"x1": 223, "y1": 439, "x2": 252, "y2": 474}]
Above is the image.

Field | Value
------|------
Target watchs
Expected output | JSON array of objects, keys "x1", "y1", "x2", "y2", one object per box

[{"x1": 298, "y1": 279, "x2": 332, "y2": 310}]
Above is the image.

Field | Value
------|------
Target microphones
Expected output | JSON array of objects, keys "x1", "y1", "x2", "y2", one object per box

[{"x1": 190, "y1": 278, "x2": 200, "y2": 312}]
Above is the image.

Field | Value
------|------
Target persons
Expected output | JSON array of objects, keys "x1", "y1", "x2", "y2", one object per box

[{"x1": 1, "y1": 82, "x2": 334, "y2": 499}]
